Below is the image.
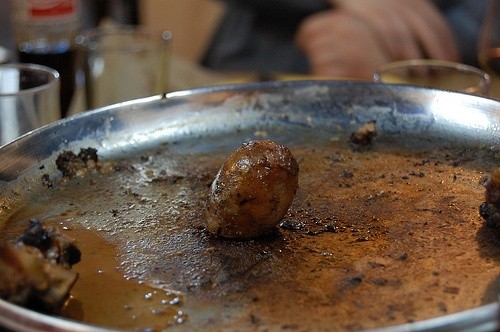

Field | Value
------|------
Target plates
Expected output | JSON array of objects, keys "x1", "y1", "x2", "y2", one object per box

[{"x1": 0, "y1": 81, "x2": 500, "y2": 332}]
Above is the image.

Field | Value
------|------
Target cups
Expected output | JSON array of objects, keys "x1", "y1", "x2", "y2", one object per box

[
  {"x1": 375, "y1": 61, "x2": 490, "y2": 94},
  {"x1": 1, "y1": 16, "x2": 79, "y2": 117},
  {"x1": 0, "y1": 63, "x2": 60, "y2": 143},
  {"x1": 80, "y1": 28, "x2": 170, "y2": 110}
]
